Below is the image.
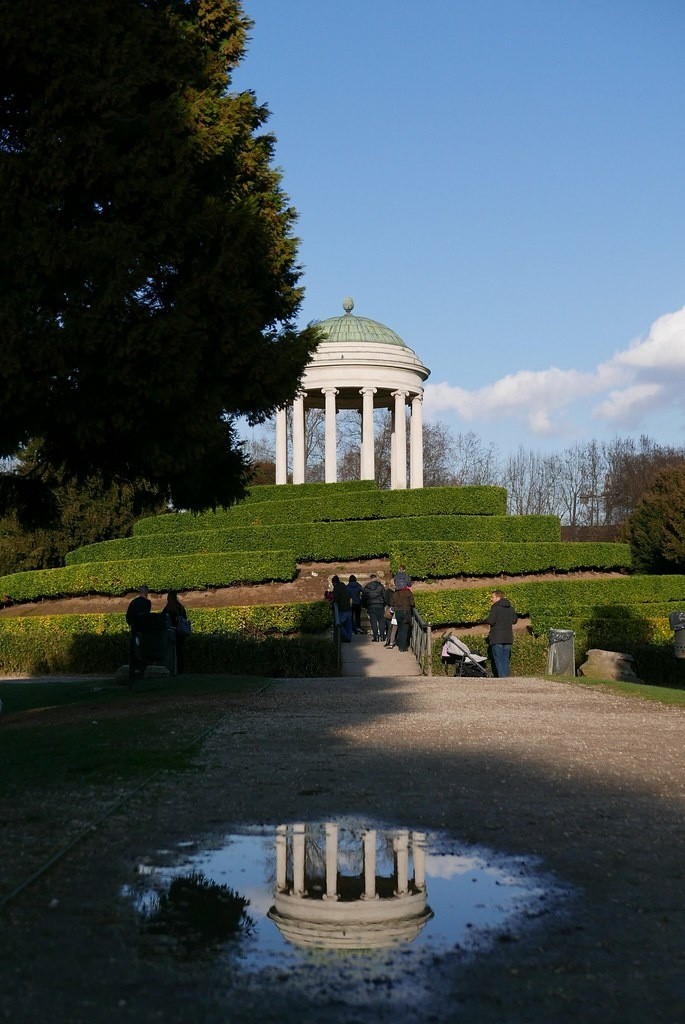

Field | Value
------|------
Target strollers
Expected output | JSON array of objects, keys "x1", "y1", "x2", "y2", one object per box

[{"x1": 440, "y1": 631, "x2": 494, "y2": 678}]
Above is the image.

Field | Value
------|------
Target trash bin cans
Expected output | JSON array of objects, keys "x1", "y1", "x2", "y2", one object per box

[
  {"x1": 139, "y1": 611, "x2": 175, "y2": 672},
  {"x1": 669, "y1": 612, "x2": 685, "y2": 658},
  {"x1": 547, "y1": 629, "x2": 575, "y2": 677}
]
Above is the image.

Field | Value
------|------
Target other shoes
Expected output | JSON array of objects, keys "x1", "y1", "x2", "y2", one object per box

[
  {"x1": 341, "y1": 627, "x2": 364, "y2": 642},
  {"x1": 371, "y1": 636, "x2": 408, "y2": 652}
]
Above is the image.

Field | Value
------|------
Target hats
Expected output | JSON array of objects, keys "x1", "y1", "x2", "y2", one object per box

[
  {"x1": 349, "y1": 575, "x2": 357, "y2": 582},
  {"x1": 333, "y1": 575, "x2": 340, "y2": 583}
]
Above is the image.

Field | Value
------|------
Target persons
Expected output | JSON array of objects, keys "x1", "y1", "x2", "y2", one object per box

[
  {"x1": 164, "y1": 592, "x2": 186, "y2": 675},
  {"x1": 362, "y1": 574, "x2": 386, "y2": 642},
  {"x1": 331, "y1": 575, "x2": 352, "y2": 643},
  {"x1": 126, "y1": 586, "x2": 152, "y2": 671},
  {"x1": 384, "y1": 572, "x2": 414, "y2": 652},
  {"x1": 485, "y1": 590, "x2": 518, "y2": 678},
  {"x1": 345, "y1": 575, "x2": 364, "y2": 635}
]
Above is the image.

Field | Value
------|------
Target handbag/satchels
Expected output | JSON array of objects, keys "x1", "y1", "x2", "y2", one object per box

[{"x1": 384, "y1": 606, "x2": 393, "y2": 619}]
